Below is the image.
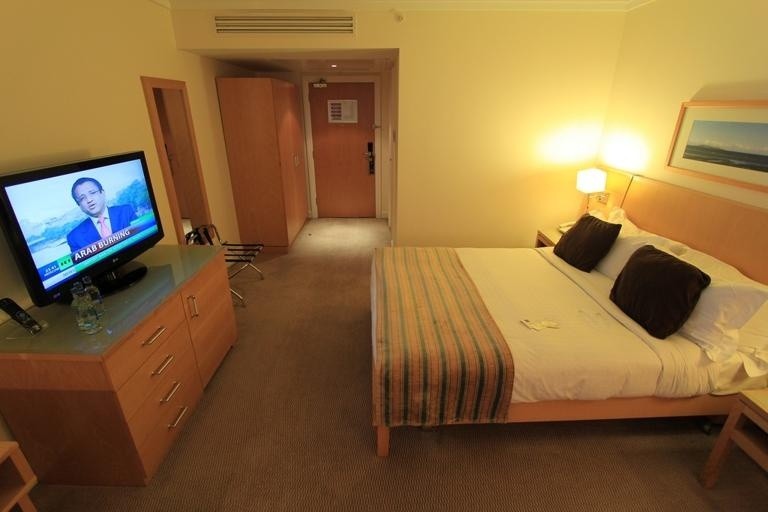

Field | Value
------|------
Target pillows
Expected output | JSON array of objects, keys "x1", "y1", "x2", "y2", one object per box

[
  {"x1": 609, "y1": 244, "x2": 711, "y2": 338},
  {"x1": 680, "y1": 279, "x2": 767, "y2": 361},
  {"x1": 595, "y1": 210, "x2": 688, "y2": 281},
  {"x1": 554, "y1": 213, "x2": 621, "y2": 270}
]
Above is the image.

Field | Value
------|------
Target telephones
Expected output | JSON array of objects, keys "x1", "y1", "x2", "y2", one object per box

[{"x1": 558, "y1": 221, "x2": 576, "y2": 234}]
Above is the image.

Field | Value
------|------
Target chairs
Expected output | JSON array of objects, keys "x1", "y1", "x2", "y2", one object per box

[{"x1": 186, "y1": 225, "x2": 264, "y2": 308}]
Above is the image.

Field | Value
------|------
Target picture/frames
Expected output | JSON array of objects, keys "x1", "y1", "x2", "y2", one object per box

[{"x1": 664, "y1": 97, "x2": 768, "y2": 196}]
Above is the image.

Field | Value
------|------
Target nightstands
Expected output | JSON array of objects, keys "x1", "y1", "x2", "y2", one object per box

[
  {"x1": 535, "y1": 226, "x2": 562, "y2": 248},
  {"x1": 697, "y1": 390, "x2": 768, "y2": 488}
]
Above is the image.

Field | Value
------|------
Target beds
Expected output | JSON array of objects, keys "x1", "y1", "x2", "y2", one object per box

[{"x1": 370, "y1": 174, "x2": 768, "y2": 460}]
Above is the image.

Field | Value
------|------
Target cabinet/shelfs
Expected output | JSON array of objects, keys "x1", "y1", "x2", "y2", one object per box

[
  {"x1": 0, "y1": 244, "x2": 240, "y2": 490},
  {"x1": 215, "y1": 77, "x2": 306, "y2": 254},
  {"x1": 0, "y1": 440, "x2": 39, "y2": 512}
]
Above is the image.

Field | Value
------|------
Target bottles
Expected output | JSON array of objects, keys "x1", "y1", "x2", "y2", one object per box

[{"x1": 69, "y1": 273, "x2": 106, "y2": 337}]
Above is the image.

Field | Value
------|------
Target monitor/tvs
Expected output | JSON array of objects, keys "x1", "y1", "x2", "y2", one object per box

[{"x1": 0, "y1": 150, "x2": 164, "y2": 308}]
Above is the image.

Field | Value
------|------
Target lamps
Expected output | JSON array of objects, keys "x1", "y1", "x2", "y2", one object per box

[{"x1": 577, "y1": 168, "x2": 605, "y2": 219}]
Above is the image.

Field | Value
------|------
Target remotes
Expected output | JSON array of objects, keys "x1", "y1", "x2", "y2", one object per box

[{"x1": 0, "y1": 298, "x2": 42, "y2": 336}]
